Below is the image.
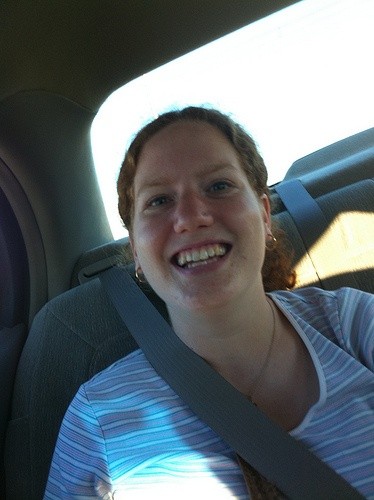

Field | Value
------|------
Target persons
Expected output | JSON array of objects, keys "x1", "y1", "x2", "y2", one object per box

[{"x1": 43, "y1": 106, "x2": 374, "y2": 500}]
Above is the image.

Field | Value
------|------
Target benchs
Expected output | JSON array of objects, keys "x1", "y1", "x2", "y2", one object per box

[{"x1": 6, "y1": 173, "x2": 374, "y2": 500}]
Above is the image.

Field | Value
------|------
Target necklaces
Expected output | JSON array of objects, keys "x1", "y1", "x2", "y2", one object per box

[{"x1": 238, "y1": 297, "x2": 276, "y2": 408}]
